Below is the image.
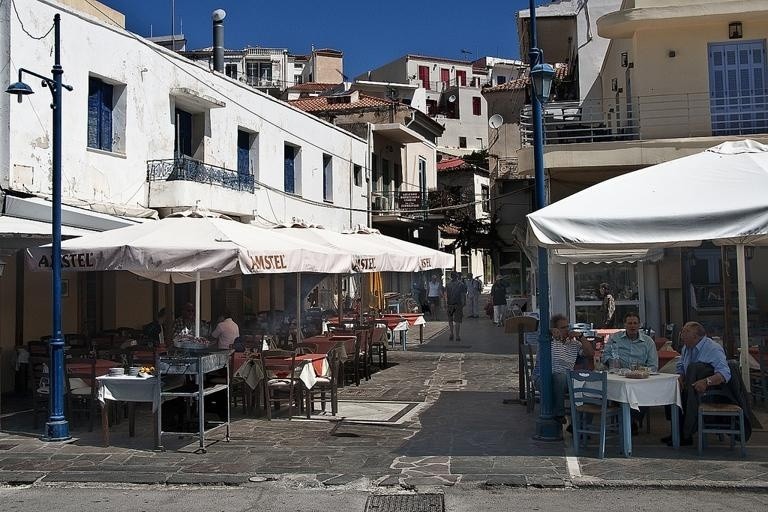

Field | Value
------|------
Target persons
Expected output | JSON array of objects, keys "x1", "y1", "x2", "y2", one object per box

[
  {"x1": 599, "y1": 283, "x2": 616, "y2": 329},
  {"x1": 413, "y1": 271, "x2": 510, "y2": 342},
  {"x1": 600, "y1": 312, "x2": 659, "y2": 436},
  {"x1": 660, "y1": 321, "x2": 732, "y2": 447},
  {"x1": 531, "y1": 314, "x2": 595, "y2": 440},
  {"x1": 142, "y1": 301, "x2": 240, "y2": 352}
]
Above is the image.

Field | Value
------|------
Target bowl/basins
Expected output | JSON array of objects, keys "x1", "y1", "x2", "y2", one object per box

[
  {"x1": 109, "y1": 367, "x2": 125, "y2": 378},
  {"x1": 129, "y1": 366, "x2": 140, "y2": 376}
]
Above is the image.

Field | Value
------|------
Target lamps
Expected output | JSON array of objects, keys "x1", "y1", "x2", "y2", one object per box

[
  {"x1": 730, "y1": 22, "x2": 744, "y2": 40},
  {"x1": 745, "y1": 246, "x2": 756, "y2": 259},
  {"x1": 611, "y1": 78, "x2": 618, "y2": 91},
  {"x1": 620, "y1": 51, "x2": 629, "y2": 67}
]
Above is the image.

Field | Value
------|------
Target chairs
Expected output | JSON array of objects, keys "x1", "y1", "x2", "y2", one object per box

[
  {"x1": 520, "y1": 327, "x2": 767, "y2": 459},
  {"x1": 556, "y1": 107, "x2": 583, "y2": 143}
]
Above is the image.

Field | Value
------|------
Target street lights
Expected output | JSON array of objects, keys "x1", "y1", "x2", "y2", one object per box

[
  {"x1": 529, "y1": 0, "x2": 565, "y2": 441},
  {"x1": 4, "y1": 13, "x2": 95, "y2": 442}
]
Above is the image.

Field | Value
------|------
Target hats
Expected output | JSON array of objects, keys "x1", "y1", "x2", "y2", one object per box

[{"x1": 496, "y1": 275, "x2": 504, "y2": 281}]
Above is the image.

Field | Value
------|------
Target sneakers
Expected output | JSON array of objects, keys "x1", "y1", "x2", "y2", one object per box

[
  {"x1": 456, "y1": 336, "x2": 460, "y2": 340},
  {"x1": 474, "y1": 315, "x2": 479, "y2": 318},
  {"x1": 468, "y1": 315, "x2": 473, "y2": 318},
  {"x1": 661, "y1": 435, "x2": 694, "y2": 447},
  {"x1": 449, "y1": 334, "x2": 453, "y2": 339}
]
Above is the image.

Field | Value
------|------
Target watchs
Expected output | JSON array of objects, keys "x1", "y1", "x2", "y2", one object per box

[{"x1": 706, "y1": 377, "x2": 712, "y2": 387}]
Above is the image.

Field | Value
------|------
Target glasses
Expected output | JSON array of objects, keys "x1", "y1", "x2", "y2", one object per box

[
  {"x1": 560, "y1": 325, "x2": 569, "y2": 329},
  {"x1": 187, "y1": 309, "x2": 195, "y2": 313}
]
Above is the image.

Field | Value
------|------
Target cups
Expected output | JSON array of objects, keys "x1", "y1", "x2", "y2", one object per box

[{"x1": 630, "y1": 359, "x2": 638, "y2": 372}]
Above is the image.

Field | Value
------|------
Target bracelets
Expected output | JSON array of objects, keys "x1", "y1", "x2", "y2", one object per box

[{"x1": 578, "y1": 332, "x2": 584, "y2": 339}]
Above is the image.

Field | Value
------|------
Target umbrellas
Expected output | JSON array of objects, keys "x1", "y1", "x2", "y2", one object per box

[
  {"x1": 524, "y1": 138, "x2": 768, "y2": 405},
  {"x1": 243, "y1": 216, "x2": 390, "y2": 344},
  {"x1": 22, "y1": 199, "x2": 353, "y2": 340},
  {"x1": 336, "y1": 225, "x2": 456, "y2": 328}
]
